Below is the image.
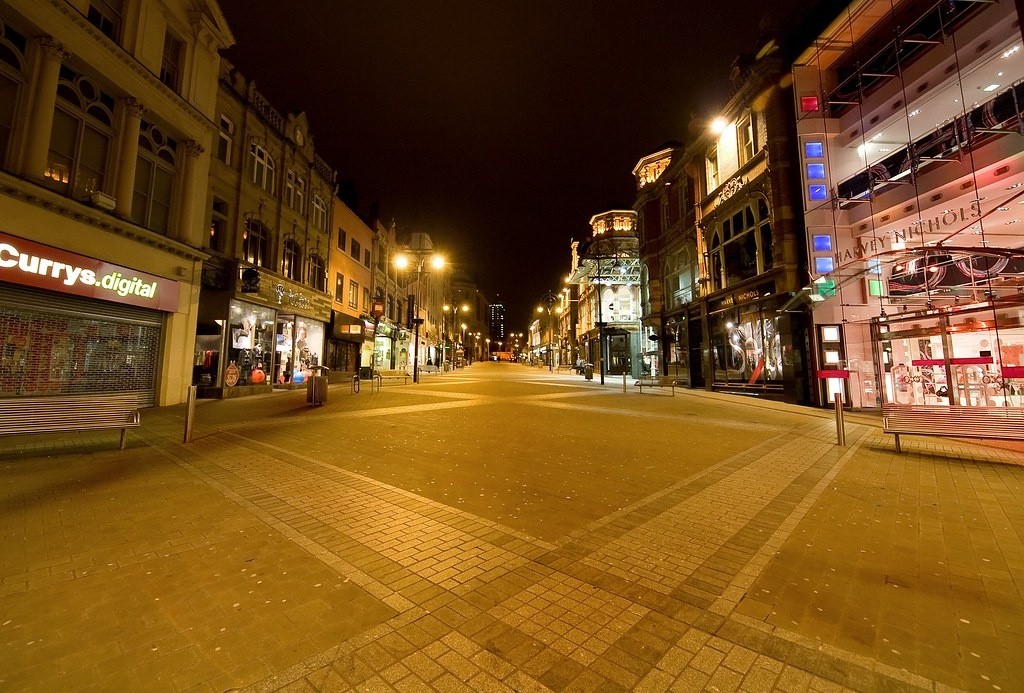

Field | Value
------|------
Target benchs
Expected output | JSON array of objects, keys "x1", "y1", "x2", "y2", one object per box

[
  {"x1": 418, "y1": 366, "x2": 439, "y2": 376},
  {"x1": 634, "y1": 375, "x2": 678, "y2": 397},
  {"x1": 0, "y1": 395, "x2": 140, "y2": 450},
  {"x1": 555, "y1": 365, "x2": 573, "y2": 375},
  {"x1": 882, "y1": 402, "x2": 1024, "y2": 454},
  {"x1": 374, "y1": 370, "x2": 411, "y2": 387}
]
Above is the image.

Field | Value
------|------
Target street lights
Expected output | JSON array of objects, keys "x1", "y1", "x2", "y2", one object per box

[
  {"x1": 536, "y1": 305, "x2": 564, "y2": 372},
  {"x1": 442, "y1": 303, "x2": 469, "y2": 370},
  {"x1": 577, "y1": 233, "x2": 622, "y2": 385},
  {"x1": 393, "y1": 250, "x2": 446, "y2": 382},
  {"x1": 510, "y1": 332, "x2": 523, "y2": 364}
]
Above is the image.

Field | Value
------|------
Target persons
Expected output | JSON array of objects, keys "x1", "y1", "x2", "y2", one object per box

[
  {"x1": 426, "y1": 357, "x2": 432, "y2": 373},
  {"x1": 511, "y1": 353, "x2": 515, "y2": 361},
  {"x1": 576, "y1": 355, "x2": 585, "y2": 375},
  {"x1": 311, "y1": 353, "x2": 318, "y2": 373},
  {"x1": 279, "y1": 323, "x2": 307, "y2": 348}
]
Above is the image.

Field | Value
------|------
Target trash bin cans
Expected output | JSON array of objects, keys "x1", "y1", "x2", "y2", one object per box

[
  {"x1": 538, "y1": 360, "x2": 542, "y2": 368},
  {"x1": 444, "y1": 360, "x2": 450, "y2": 371},
  {"x1": 585, "y1": 364, "x2": 594, "y2": 382},
  {"x1": 306, "y1": 365, "x2": 330, "y2": 407}
]
